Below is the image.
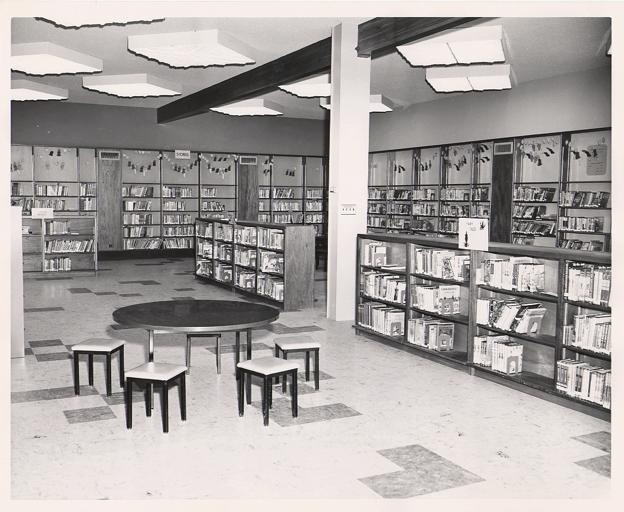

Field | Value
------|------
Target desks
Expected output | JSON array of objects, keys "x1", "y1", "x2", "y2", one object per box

[{"x1": 110, "y1": 297, "x2": 279, "y2": 382}]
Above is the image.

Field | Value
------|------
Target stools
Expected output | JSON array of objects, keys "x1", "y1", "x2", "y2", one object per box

[{"x1": 70, "y1": 331, "x2": 320, "y2": 434}]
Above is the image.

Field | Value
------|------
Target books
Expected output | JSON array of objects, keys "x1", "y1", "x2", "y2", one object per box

[
  {"x1": 409, "y1": 276, "x2": 460, "y2": 314},
  {"x1": 556, "y1": 359, "x2": 611, "y2": 408},
  {"x1": 562, "y1": 303, "x2": 611, "y2": 355},
  {"x1": 408, "y1": 316, "x2": 454, "y2": 351},
  {"x1": 11, "y1": 182, "x2": 96, "y2": 216},
  {"x1": 564, "y1": 261, "x2": 610, "y2": 307},
  {"x1": 472, "y1": 335, "x2": 523, "y2": 374},
  {"x1": 476, "y1": 259, "x2": 545, "y2": 293},
  {"x1": 475, "y1": 298, "x2": 547, "y2": 334},
  {"x1": 123, "y1": 184, "x2": 230, "y2": 250},
  {"x1": 360, "y1": 239, "x2": 406, "y2": 271},
  {"x1": 21, "y1": 225, "x2": 30, "y2": 234},
  {"x1": 366, "y1": 187, "x2": 488, "y2": 237},
  {"x1": 359, "y1": 297, "x2": 405, "y2": 337},
  {"x1": 196, "y1": 220, "x2": 285, "y2": 302},
  {"x1": 360, "y1": 267, "x2": 406, "y2": 304},
  {"x1": 515, "y1": 182, "x2": 607, "y2": 254},
  {"x1": 410, "y1": 243, "x2": 470, "y2": 282},
  {"x1": 43, "y1": 220, "x2": 93, "y2": 271},
  {"x1": 259, "y1": 185, "x2": 324, "y2": 237}
]
